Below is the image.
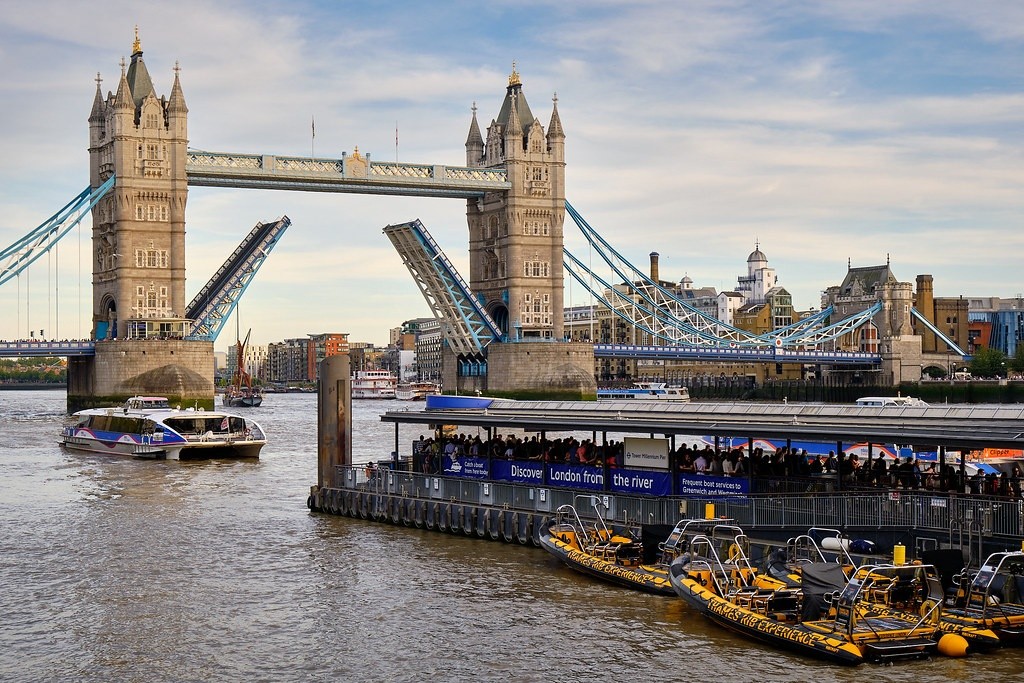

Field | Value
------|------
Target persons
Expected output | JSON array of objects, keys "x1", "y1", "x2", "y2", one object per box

[
  {"x1": 0, "y1": 335, "x2": 183, "y2": 343},
  {"x1": 672, "y1": 444, "x2": 1024, "y2": 526},
  {"x1": 225, "y1": 390, "x2": 260, "y2": 401},
  {"x1": 367, "y1": 461, "x2": 382, "y2": 480},
  {"x1": 412, "y1": 433, "x2": 627, "y2": 494},
  {"x1": 941, "y1": 374, "x2": 1024, "y2": 381}
]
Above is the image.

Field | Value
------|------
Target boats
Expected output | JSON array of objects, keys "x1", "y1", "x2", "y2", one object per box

[
  {"x1": 261, "y1": 385, "x2": 319, "y2": 393},
  {"x1": 350, "y1": 357, "x2": 442, "y2": 402},
  {"x1": 537, "y1": 495, "x2": 719, "y2": 599},
  {"x1": 595, "y1": 381, "x2": 691, "y2": 403},
  {"x1": 56, "y1": 392, "x2": 268, "y2": 462},
  {"x1": 221, "y1": 384, "x2": 264, "y2": 408},
  {"x1": 855, "y1": 391, "x2": 931, "y2": 407},
  {"x1": 656, "y1": 498, "x2": 1024, "y2": 669}
]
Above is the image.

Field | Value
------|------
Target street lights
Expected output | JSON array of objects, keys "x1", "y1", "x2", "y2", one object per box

[
  {"x1": 919, "y1": 363, "x2": 924, "y2": 381},
  {"x1": 952, "y1": 362, "x2": 957, "y2": 380},
  {"x1": 1000, "y1": 362, "x2": 1006, "y2": 379}
]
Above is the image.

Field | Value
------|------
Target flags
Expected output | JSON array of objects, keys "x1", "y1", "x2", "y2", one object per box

[
  {"x1": 220, "y1": 417, "x2": 230, "y2": 430},
  {"x1": 395, "y1": 124, "x2": 399, "y2": 146},
  {"x1": 312, "y1": 118, "x2": 315, "y2": 138}
]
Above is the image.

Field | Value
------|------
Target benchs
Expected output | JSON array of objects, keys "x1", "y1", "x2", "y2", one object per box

[
  {"x1": 857, "y1": 579, "x2": 894, "y2": 604},
  {"x1": 588, "y1": 542, "x2": 640, "y2": 565},
  {"x1": 725, "y1": 589, "x2": 800, "y2": 619}
]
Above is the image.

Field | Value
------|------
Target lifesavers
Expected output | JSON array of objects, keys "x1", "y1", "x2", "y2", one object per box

[
  {"x1": 729, "y1": 544, "x2": 740, "y2": 561},
  {"x1": 307, "y1": 496, "x2": 314, "y2": 508},
  {"x1": 244, "y1": 428, "x2": 250, "y2": 436},
  {"x1": 920, "y1": 599, "x2": 939, "y2": 623}
]
